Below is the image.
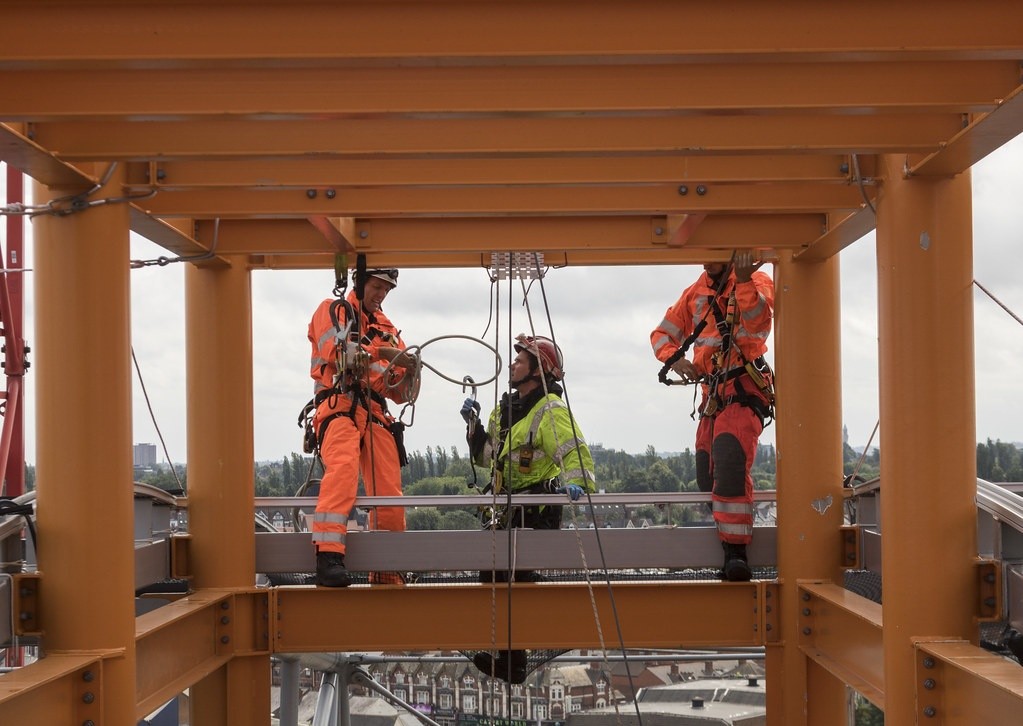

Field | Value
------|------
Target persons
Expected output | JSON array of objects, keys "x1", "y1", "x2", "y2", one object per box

[
  {"x1": 650, "y1": 253, "x2": 776, "y2": 583},
  {"x1": 307, "y1": 269, "x2": 423, "y2": 587},
  {"x1": 460, "y1": 333, "x2": 596, "y2": 685}
]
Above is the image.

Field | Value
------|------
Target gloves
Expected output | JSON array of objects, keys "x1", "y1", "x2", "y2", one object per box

[
  {"x1": 460, "y1": 398, "x2": 481, "y2": 425},
  {"x1": 555, "y1": 485, "x2": 585, "y2": 501}
]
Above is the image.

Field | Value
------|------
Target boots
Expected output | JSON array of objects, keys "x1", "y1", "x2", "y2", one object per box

[
  {"x1": 474, "y1": 652, "x2": 527, "y2": 683},
  {"x1": 721, "y1": 541, "x2": 753, "y2": 582},
  {"x1": 316, "y1": 552, "x2": 353, "y2": 587}
]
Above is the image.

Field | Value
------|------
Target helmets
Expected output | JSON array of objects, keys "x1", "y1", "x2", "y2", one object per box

[
  {"x1": 351, "y1": 267, "x2": 397, "y2": 289},
  {"x1": 515, "y1": 337, "x2": 564, "y2": 382}
]
